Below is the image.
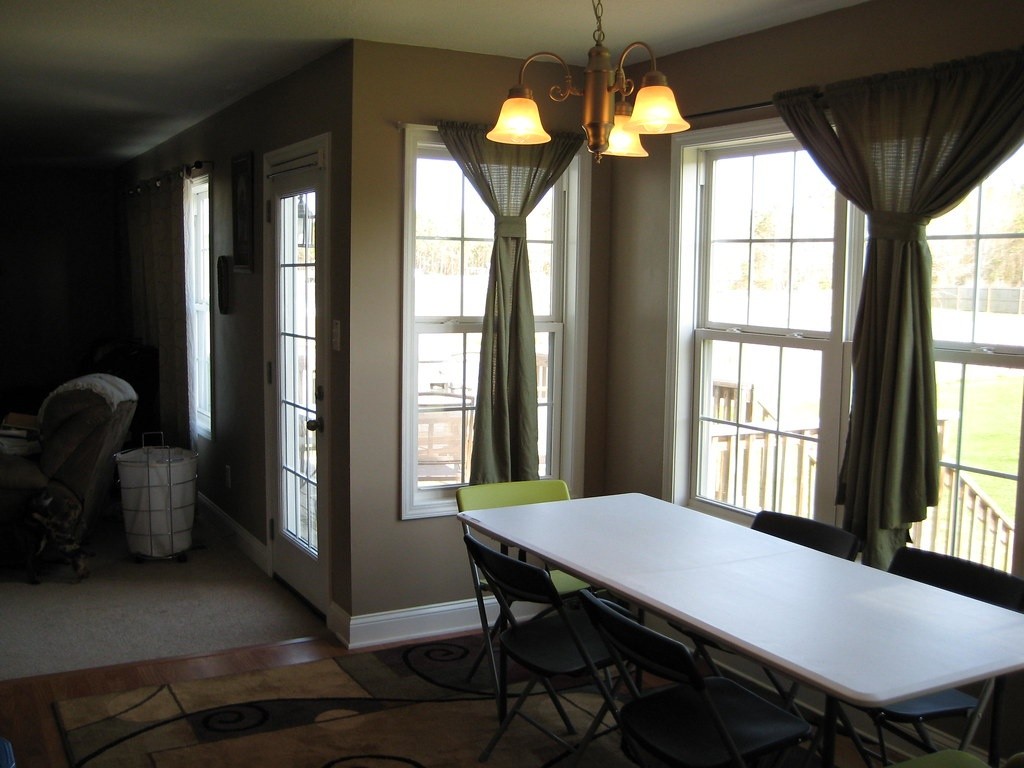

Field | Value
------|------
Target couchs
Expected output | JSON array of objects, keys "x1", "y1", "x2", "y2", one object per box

[{"x1": 0, "y1": 371, "x2": 138, "y2": 583}]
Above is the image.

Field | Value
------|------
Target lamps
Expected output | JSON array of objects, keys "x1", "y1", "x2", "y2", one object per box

[{"x1": 486, "y1": 0, "x2": 690, "y2": 164}]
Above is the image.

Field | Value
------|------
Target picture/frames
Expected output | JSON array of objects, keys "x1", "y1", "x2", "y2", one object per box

[{"x1": 231, "y1": 150, "x2": 255, "y2": 275}]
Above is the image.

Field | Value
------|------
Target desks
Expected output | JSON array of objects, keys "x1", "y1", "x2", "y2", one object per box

[{"x1": 455, "y1": 491, "x2": 1024, "y2": 768}]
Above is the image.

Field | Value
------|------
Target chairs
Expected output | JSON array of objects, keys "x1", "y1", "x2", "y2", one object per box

[
  {"x1": 578, "y1": 588, "x2": 815, "y2": 768},
  {"x1": 457, "y1": 479, "x2": 592, "y2": 686},
  {"x1": 667, "y1": 511, "x2": 860, "y2": 758},
  {"x1": 886, "y1": 749, "x2": 1024, "y2": 768},
  {"x1": 820, "y1": 546, "x2": 1024, "y2": 768},
  {"x1": 462, "y1": 534, "x2": 641, "y2": 768}
]
